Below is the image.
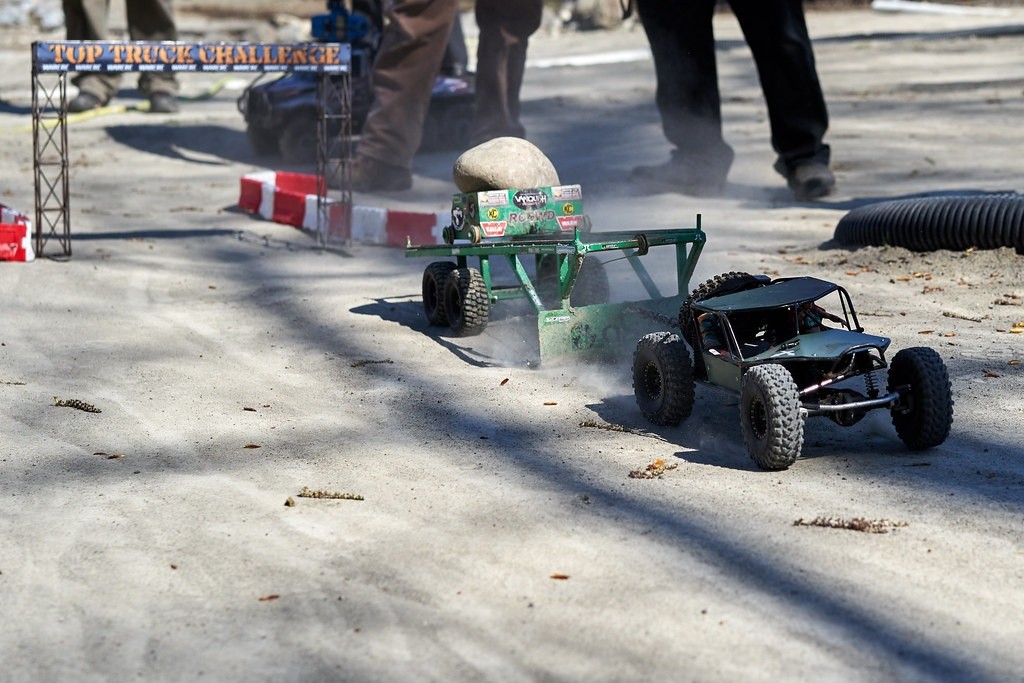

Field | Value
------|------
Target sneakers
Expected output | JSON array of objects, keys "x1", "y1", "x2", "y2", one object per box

[
  {"x1": 786, "y1": 161, "x2": 835, "y2": 201},
  {"x1": 319, "y1": 154, "x2": 413, "y2": 197},
  {"x1": 633, "y1": 162, "x2": 726, "y2": 197}
]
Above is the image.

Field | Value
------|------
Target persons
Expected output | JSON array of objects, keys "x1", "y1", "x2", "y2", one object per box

[
  {"x1": 629, "y1": 0, "x2": 836, "y2": 200},
  {"x1": 63, "y1": 0, "x2": 180, "y2": 112},
  {"x1": 323, "y1": 1, "x2": 546, "y2": 193}
]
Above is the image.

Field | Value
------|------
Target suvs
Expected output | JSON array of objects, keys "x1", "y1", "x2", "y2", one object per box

[{"x1": 631, "y1": 269, "x2": 955, "y2": 472}]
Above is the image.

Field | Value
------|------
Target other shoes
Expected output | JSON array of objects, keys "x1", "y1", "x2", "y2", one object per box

[
  {"x1": 67, "y1": 91, "x2": 110, "y2": 112},
  {"x1": 149, "y1": 91, "x2": 178, "y2": 113}
]
